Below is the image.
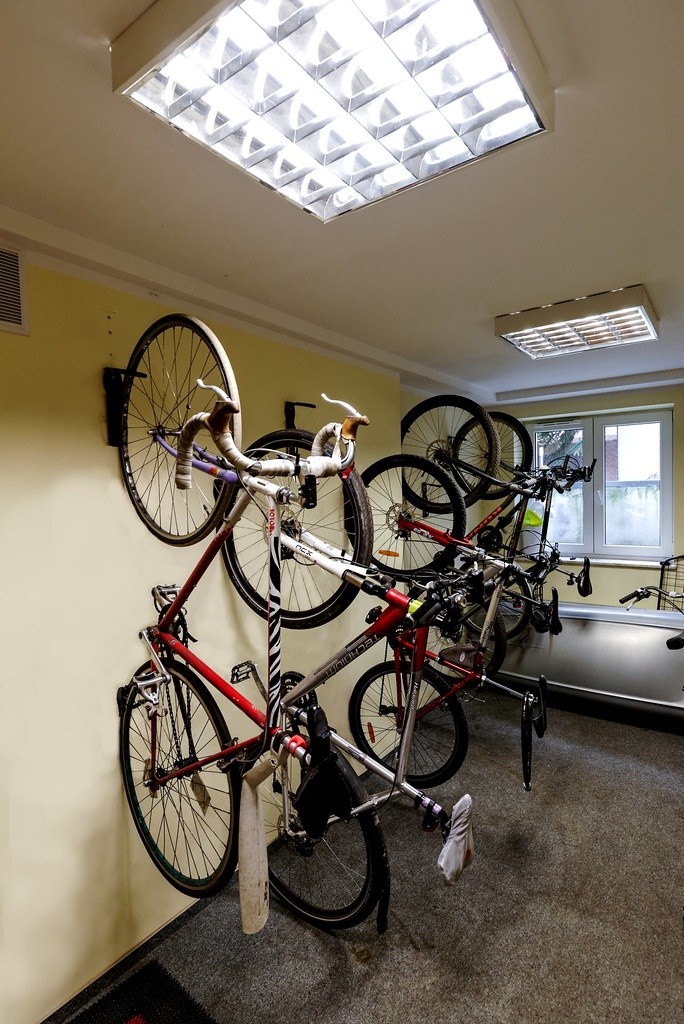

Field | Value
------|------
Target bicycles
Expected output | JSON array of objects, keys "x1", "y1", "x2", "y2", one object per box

[
  {"x1": 618, "y1": 585, "x2": 684, "y2": 651},
  {"x1": 118, "y1": 311, "x2": 598, "y2": 937}
]
